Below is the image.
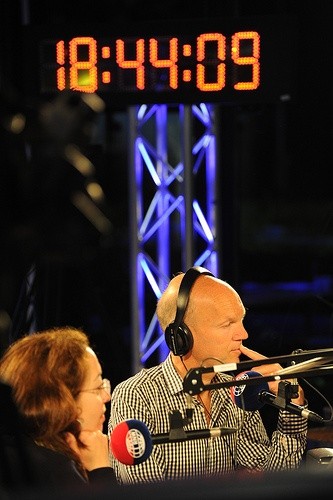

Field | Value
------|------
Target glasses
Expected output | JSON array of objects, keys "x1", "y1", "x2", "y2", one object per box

[{"x1": 79, "y1": 378, "x2": 111, "y2": 396}]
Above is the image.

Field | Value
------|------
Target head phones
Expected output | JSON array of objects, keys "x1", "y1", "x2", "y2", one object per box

[{"x1": 165, "y1": 266, "x2": 215, "y2": 356}]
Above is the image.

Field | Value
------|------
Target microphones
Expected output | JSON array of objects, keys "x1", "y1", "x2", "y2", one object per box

[
  {"x1": 110, "y1": 419, "x2": 237, "y2": 465},
  {"x1": 230, "y1": 370, "x2": 323, "y2": 422}
]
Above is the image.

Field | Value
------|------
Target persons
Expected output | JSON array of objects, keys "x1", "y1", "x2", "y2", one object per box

[
  {"x1": 107, "y1": 273, "x2": 309, "y2": 485},
  {"x1": 0, "y1": 330, "x2": 119, "y2": 500}
]
